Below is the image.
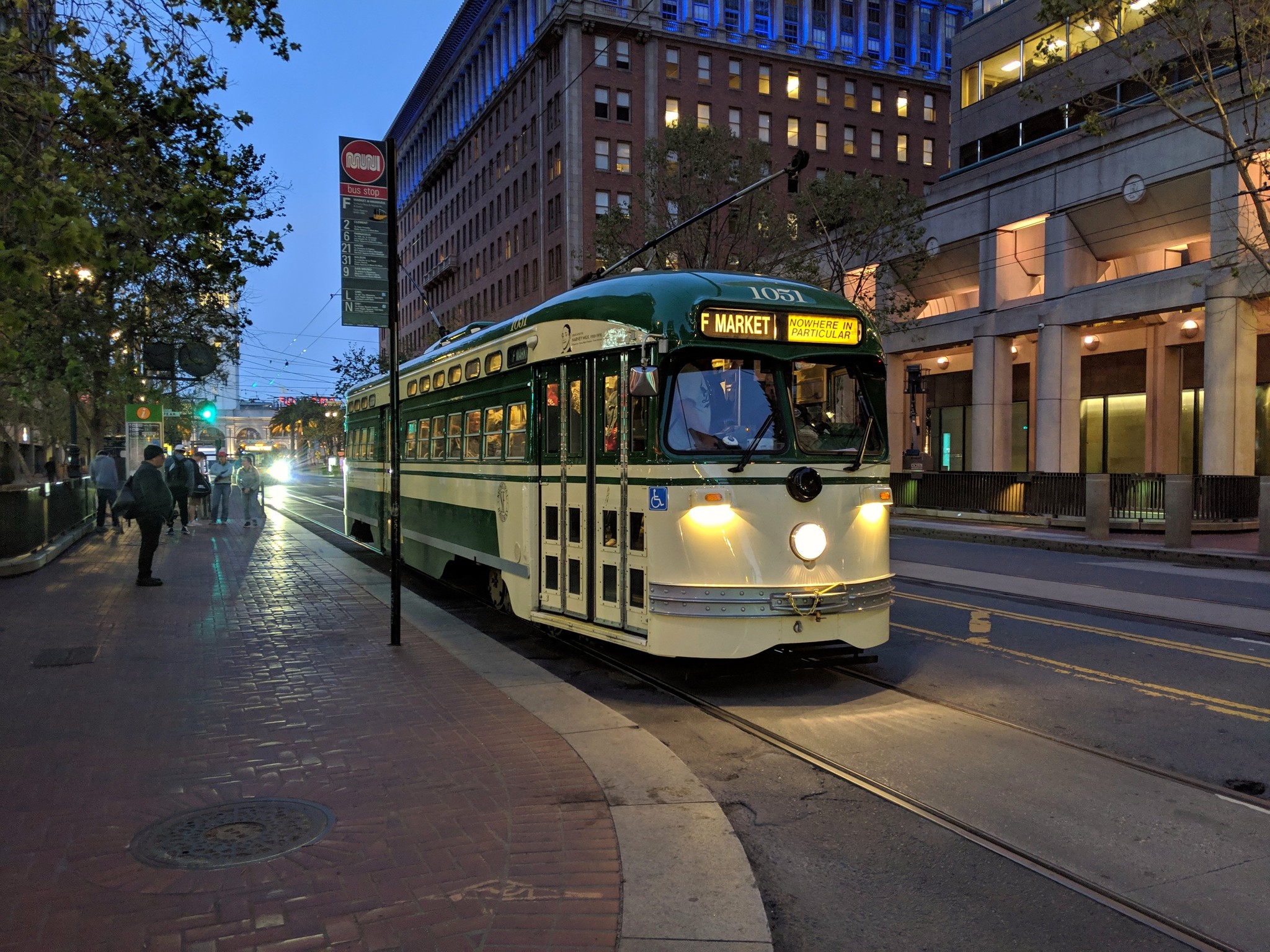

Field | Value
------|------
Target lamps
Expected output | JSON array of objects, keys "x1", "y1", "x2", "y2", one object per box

[
  {"x1": 1085, "y1": 335, "x2": 1100, "y2": 350},
  {"x1": 1180, "y1": 322, "x2": 1198, "y2": 338},
  {"x1": 937, "y1": 356, "x2": 949, "y2": 369},
  {"x1": 1011, "y1": 347, "x2": 1018, "y2": 360}
]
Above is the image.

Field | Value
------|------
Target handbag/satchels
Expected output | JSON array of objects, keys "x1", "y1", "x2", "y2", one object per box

[{"x1": 194, "y1": 477, "x2": 211, "y2": 495}]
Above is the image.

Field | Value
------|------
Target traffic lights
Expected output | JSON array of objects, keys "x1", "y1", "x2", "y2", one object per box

[{"x1": 196, "y1": 400, "x2": 217, "y2": 421}]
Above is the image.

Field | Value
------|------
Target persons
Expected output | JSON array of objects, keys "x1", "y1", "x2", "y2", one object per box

[
  {"x1": 188, "y1": 451, "x2": 211, "y2": 528},
  {"x1": 89, "y1": 450, "x2": 122, "y2": 534},
  {"x1": 165, "y1": 444, "x2": 194, "y2": 535},
  {"x1": 408, "y1": 378, "x2": 619, "y2": 459},
  {"x1": 43, "y1": 457, "x2": 57, "y2": 483},
  {"x1": 111, "y1": 444, "x2": 174, "y2": 590},
  {"x1": 236, "y1": 458, "x2": 261, "y2": 528},
  {"x1": 794, "y1": 381, "x2": 874, "y2": 451},
  {"x1": 667, "y1": 358, "x2": 822, "y2": 452},
  {"x1": 208, "y1": 451, "x2": 232, "y2": 527}
]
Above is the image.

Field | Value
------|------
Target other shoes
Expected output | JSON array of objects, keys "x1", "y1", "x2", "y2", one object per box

[
  {"x1": 221, "y1": 520, "x2": 228, "y2": 525},
  {"x1": 166, "y1": 528, "x2": 174, "y2": 535},
  {"x1": 243, "y1": 521, "x2": 251, "y2": 527},
  {"x1": 181, "y1": 526, "x2": 190, "y2": 534},
  {"x1": 186, "y1": 520, "x2": 202, "y2": 527},
  {"x1": 252, "y1": 519, "x2": 258, "y2": 527},
  {"x1": 135, "y1": 574, "x2": 164, "y2": 588},
  {"x1": 209, "y1": 520, "x2": 216, "y2": 524}
]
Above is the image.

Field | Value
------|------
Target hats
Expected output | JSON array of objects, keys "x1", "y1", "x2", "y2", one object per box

[
  {"x1": 217, "y1": 452, "x2": 226, "y2": 457},
  {"x1": 174, "y1": 444, "x2": 186, "y2": 451},
  {"x1": 143, "y1": 444, "x2": 163, "y2": 461}
]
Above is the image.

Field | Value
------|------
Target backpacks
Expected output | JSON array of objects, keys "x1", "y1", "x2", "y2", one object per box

[
  {"x1": 111, "y1": 475, "x2": 136, "y2": 520},
  {"x1": 168, "y1": 454, "x2": 189, "y2": 482}
]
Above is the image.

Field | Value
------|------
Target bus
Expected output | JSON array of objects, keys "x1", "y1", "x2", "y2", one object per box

[
  {"x1": 344, "y1": 149, "x2": 896, "y2": 678},
  {"x1": 238, "y1": 442, "x2": 279, "y2": 487}
]
